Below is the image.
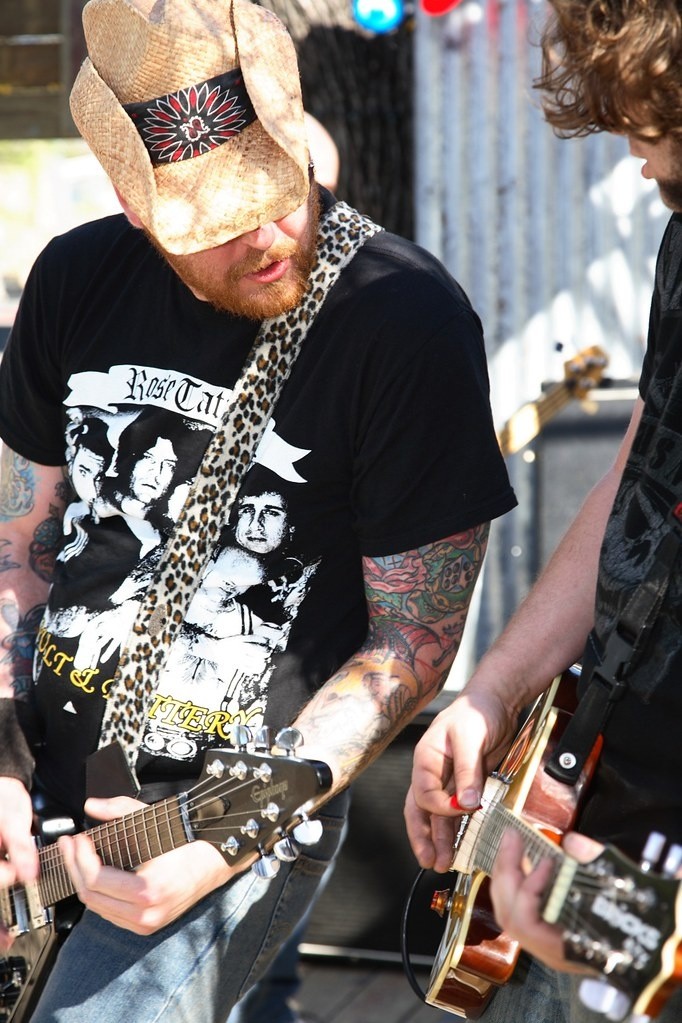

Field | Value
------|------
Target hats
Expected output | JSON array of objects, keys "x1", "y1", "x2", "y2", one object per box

[{"x1": 70, "y1": 0, "x2": 310, "y2": 256}]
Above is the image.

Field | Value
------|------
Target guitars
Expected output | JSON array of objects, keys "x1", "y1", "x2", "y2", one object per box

[
  {"x1": 422, "y1": 674, "x2": 680, "y2": 1023},
  {"x1": 0, "y1": 717, "x2": 332, "y2": 1022},
  {"x1": 492, "y1": 344, "x2": 610, "y2": 451}
]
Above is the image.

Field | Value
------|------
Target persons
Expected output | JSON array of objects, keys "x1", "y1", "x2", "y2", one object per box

[
  {"x1": 403, "y1": 0, "x2": 682, "y2": 1023},
  {"x1": 0, "y1": 0, "x2": 518, "y2": 1023},
  {"x1": 50, "y1": 412, "x2": 303, "y2": 748}
]
